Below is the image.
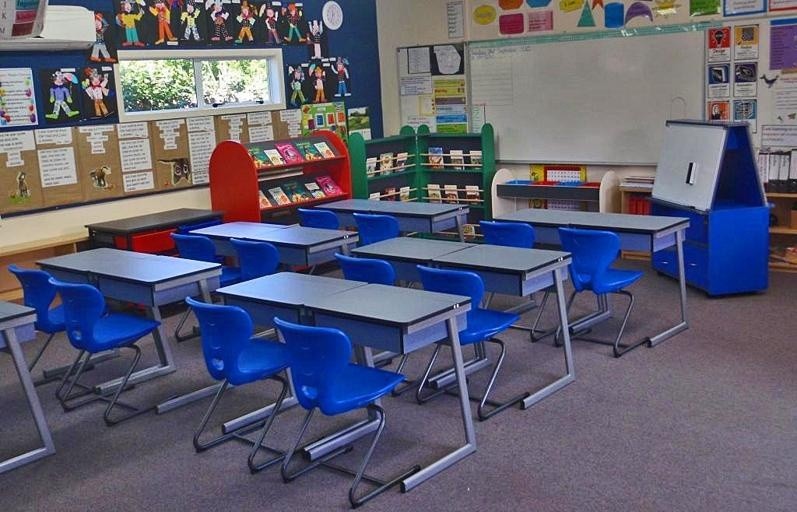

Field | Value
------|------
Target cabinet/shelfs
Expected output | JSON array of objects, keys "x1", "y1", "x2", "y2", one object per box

[{"x1": 202, "y1": 123, "x2": 496, "y2": 220}]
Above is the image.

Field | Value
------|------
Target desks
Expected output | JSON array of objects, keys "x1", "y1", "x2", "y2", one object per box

[{"x1": 0, "y1": 299, "x2": 58, "y2": 473}]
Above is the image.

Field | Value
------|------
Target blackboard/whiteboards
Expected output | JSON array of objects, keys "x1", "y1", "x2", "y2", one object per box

[
  {"x1": 469, "y1": 28, "x2": 706, "y2": 167},
  {"x1": 651, "y1": 120, "x2": 729, "y2": 212}
]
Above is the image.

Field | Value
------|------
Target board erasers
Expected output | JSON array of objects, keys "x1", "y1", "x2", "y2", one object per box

[{"x1": 686, "y1": 162, "x2": 697, "y2": 185}]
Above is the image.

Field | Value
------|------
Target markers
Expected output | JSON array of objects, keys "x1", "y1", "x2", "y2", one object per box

[{"x1": 685, "y1": 206, "x2": 695, "y2": 209}]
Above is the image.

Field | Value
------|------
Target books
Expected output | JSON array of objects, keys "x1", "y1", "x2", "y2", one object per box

[
  {"x1": 444, "y1": 184, "x2": 459, "y2": 204},
  {"x1": 395, "y1": 152, "x2": 408, "y2": 172},
  {"x1": 428, "y1": 147, "x2": 444, "y2": 169},
  {"x1": 379, "y1": 153, "x2": 393, "y2": 176},
  {"x1": 366, "y1": 157, "x2": 377, "y2": 178},
  {"x1": 384, "y1": 187, "x2": 397, "y2": 201},
  {"x1": 450, "y1": 150, "x2": 465, "y2": 170},
  {"x1": 465, "y1": 186, "x2": 481, "y2": 206},
  {"x1": 245, "y1": 138, "x2": 343, "y2": 211},
  {"x1": 620, "y1": 174, "x2": 654, "y2": 215},
  {"x1": 370, "y1": 192, "x2": 380, "y2": 201},
  {"x1": 427, "y1": 184, "x2": 442, "y2": 204},
  {"x1": 399, "y1": 186, "x2": 410, "y2": 201},
  {"x1": 469, "y1": 151, "x2": 483, "y2": 170}
]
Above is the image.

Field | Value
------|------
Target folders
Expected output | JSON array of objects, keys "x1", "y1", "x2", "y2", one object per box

[{"x1": 758, "y1": 148, "x2": 797, "y2": 193}]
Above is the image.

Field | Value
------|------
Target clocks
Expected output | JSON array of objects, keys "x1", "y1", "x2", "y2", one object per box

[{"x1": 321, "y1": 1, "x2": 344, "y2": 31}]
